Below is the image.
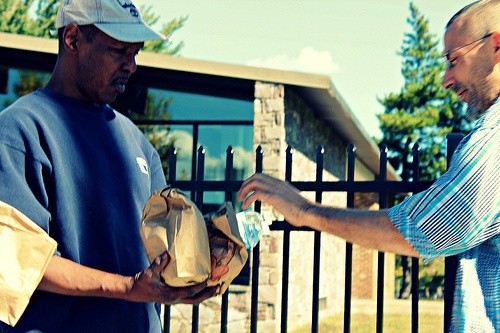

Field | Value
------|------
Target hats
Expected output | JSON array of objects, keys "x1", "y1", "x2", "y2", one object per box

[{"x1": 55, "y1": 0, "x2": 167, "y2": 43}]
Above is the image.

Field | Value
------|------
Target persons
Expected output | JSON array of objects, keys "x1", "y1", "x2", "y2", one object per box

[
  {"x1": 0, "y1": 0, "x2": 221, "y2": 333},
  {"x1": 238, "y1": 1, "x2": 500, "y2": 333}
]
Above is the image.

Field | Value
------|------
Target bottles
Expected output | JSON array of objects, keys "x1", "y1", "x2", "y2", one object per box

[
  {"x1": 235, "y1": 211, "x2": 272, "y2": 225},
  {"x1": 238, "y1": 220, "x2": 270, "y2": 249}
]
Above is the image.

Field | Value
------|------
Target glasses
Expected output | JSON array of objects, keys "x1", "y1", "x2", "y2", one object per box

[{"x1": 442, "y1": 32, "x2": 493, "y2": 68}]
